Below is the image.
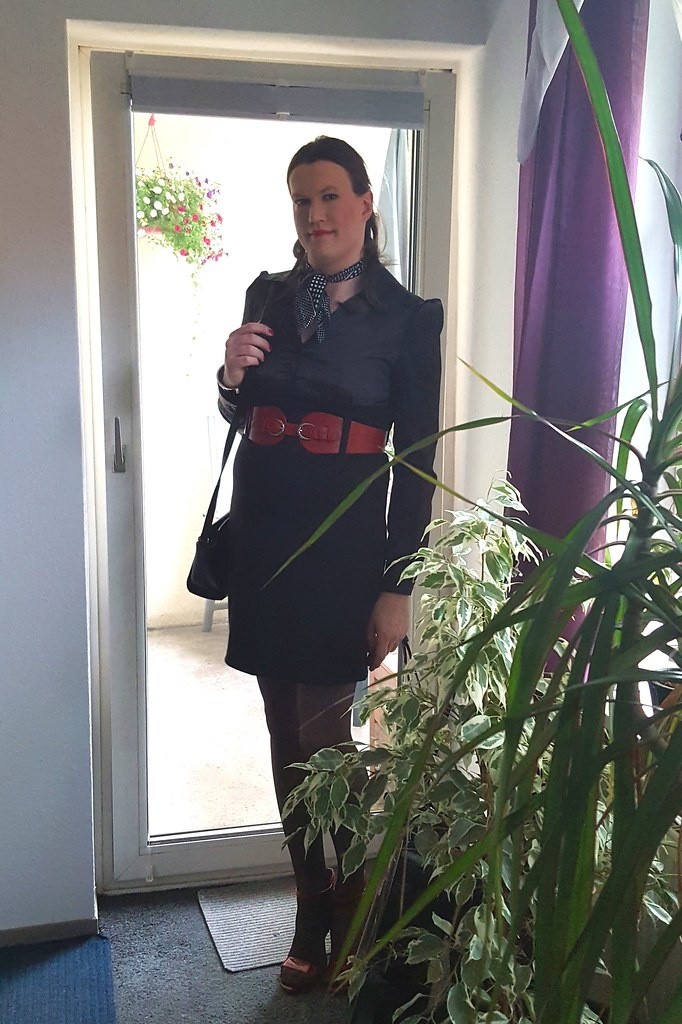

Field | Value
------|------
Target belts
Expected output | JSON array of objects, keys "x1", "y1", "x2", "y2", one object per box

[{"x1": 243, "y1": 406, "x2": 387, "y2": 454}]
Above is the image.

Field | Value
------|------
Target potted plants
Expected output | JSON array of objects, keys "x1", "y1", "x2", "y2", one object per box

[{"x1": 261, "y1": 0, "x2": 682, "y2": 1024}]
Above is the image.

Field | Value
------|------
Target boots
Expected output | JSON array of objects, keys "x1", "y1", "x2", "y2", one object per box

[
  {"x1": 280, "y1": 868, "x2": 336, "y2": 994},
  {"x1": 323, "y1": 870, "x2": 368, "y2": 993}
]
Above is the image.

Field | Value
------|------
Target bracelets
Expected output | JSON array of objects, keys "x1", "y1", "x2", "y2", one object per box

[{"x1": 216, "y1": 364, "x2": 245, "y2": 395}]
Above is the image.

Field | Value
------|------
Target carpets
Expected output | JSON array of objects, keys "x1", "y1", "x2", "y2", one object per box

[
  {"x1": 198, "y1": 875, "x2": 332, "y2": 972},
  {"x1": 0, "y1": 935, "x2": 118, "y2": 1024}
]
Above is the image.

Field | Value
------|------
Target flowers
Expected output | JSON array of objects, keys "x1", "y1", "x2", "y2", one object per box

[{"x1": 133, "y1": 157, "x2": 229, "y2": 289}]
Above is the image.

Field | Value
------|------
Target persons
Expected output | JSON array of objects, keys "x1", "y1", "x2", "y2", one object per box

[{"x1": 217, "y1": 135, "x2": 444, "y2": 992}]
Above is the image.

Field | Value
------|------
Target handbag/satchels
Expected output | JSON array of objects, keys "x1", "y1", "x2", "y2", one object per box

[{"x1": 187, "y1": 513, "x2": 230, "y2": 600}]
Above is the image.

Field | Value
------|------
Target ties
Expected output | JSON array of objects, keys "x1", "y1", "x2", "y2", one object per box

[{"x1": 294, "y1": 259, "x2": 370, "y2": 345}]
